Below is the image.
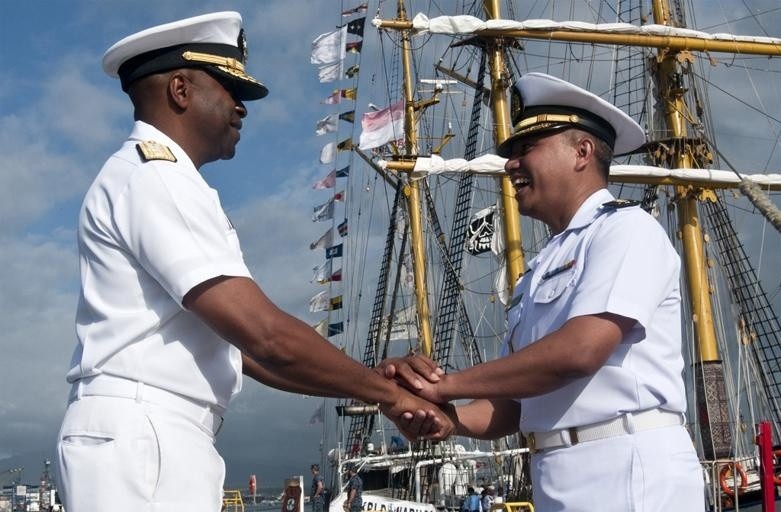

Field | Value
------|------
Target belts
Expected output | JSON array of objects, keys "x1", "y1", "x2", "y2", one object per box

[
  {"x1": 69, "y1": 378, "x2": 224, "y2": 441},
  {"x1": 528, "y1": 412, "x2": 683, "y2": 454}
]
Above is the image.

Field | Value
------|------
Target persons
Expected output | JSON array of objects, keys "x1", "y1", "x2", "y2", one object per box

[
  {"x1": 482, "y1": 489, "x2": 494, "y2": 511},
  {"x1": 53, "y1": 8, "x2": 461, "y2": 512},
  {"x1": 345, "y1": 461, "x2": 363, "y2": 512},
  {"x1": 310, "y1": 461, "x2": 326, "y2": 511},
  {"x1": 402, "y1": 69, "x2": 709, "y2": 512},
  {"x1": 461, "y1": 487, "x2": 480, "y2": 512}
]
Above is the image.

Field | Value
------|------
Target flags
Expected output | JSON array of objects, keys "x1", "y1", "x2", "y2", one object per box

[
  {"x1": 309, "y1": 2, "x2": 367, "y2": 355},
  {"x1": 359, "y1": 98, "x2": 406, "y2": 151},
  {"x1": 309, "y1": 403, "x2": 323, "y2": 425}
]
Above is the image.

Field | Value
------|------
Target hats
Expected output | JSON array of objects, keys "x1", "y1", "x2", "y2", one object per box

[
  {"x1": 102, "y1": 11, "x2": 268, "y2": 99},
  {"x1": 497, "y1": 72, "x2": 646, "y2": 157}
]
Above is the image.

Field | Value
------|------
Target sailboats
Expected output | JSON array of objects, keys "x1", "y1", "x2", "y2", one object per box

[{"x1": 314, "y1": 0, "x2": 780, "y2": 512}]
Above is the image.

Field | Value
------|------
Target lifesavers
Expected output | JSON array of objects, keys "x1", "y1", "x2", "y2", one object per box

[
  {"x1": 249, "y1": 476, "x2": 257, "y2": 495},
  {"x1": 720, "y1": 462, "x2": 747, "y2": 496},
  {"x1": 770, "y1": 450, "x2": 781, "y2": 486}
]
